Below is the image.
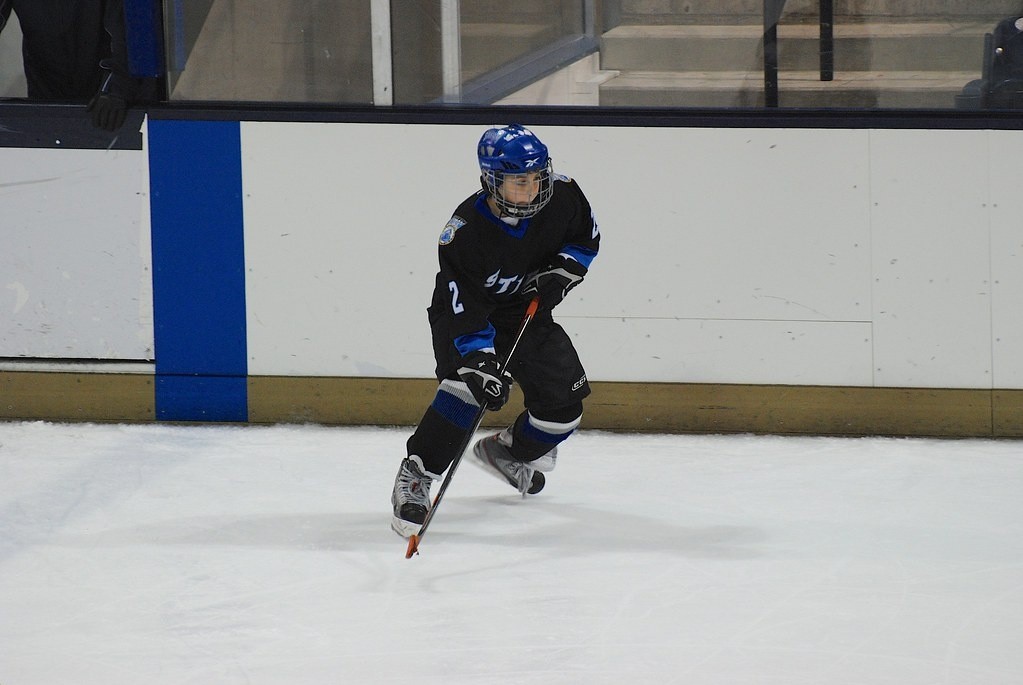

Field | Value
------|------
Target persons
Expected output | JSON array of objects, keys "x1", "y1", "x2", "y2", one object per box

[
  {"x1": 0, "y1": 0, "x2": 165, "y2": 131},
  {"x1": 390, "y1": 123, "x2": 601, "y2": 543}
]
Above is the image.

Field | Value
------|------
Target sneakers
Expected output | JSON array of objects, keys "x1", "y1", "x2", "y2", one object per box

[
  {"x1": 390, "y1": 455, "x2": 443, "y2": 537},
  {"x1": 464, "y1": 426, "x2": 559, "y2": 496}
]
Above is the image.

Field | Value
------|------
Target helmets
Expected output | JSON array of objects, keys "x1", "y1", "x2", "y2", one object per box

[{"x1": 477, "y1": 125, "x2": 554, "y2": 219}]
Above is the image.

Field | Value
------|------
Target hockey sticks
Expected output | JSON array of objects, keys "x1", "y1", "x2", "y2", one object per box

[{"x1": 405, "y1": 296, "x2": 541, "y2": 561}]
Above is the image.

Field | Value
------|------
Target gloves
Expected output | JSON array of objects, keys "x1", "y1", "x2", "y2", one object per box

[
  {"x1": 456, "y1": 350, "x2": 512, "y2": 411},
  {"x1": 85, "y1": 93, "x2": 128, "y2": 133},
  {"x1": 521, "y1": 256, "x2": 588, "y2": 315}
]
